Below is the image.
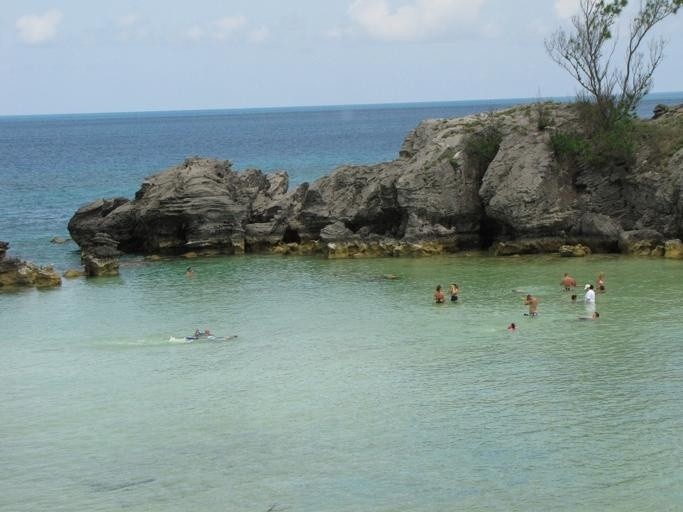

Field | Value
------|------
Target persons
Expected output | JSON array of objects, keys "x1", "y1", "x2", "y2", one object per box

[
  {"x1": 432, "y1": 284, "x2": 445, "y2": 304},
  {"x1": 203, "y1": 328, "x2": 211, "y2": 336},
  {"x1": 571, "y1": 294, "x2": 576, "y2": 301},
  {"x1": 522, "y1": 294, "x2": 537, "y2": 318},
  {"x1": 582, "y1": 301, "x2": 596, "y2": 316},
  {"x1": 507, "y1": 323, "x2": 517, "y2": 333},
  {"x1": 184, "y1": 266, "x2": 194, "y2": 279},
  {"x1": 595, "y1": 271, "x2": 605, "y2": 294},
  {"x1": 446, "y1": 283, "x2": 459, "y2": 301},
  {"x1": 576, "y1": 312, "x2": 599, "y2": 321},
  {"x1": 559, "y1": 272, "x2": 576, "y2": 292},
  {"x1": 193, "y1": 329, "x2": 200, "y2": 338},
  {"x1": 583, "y1": 283, "x2": 595, "y2": 303}
]
